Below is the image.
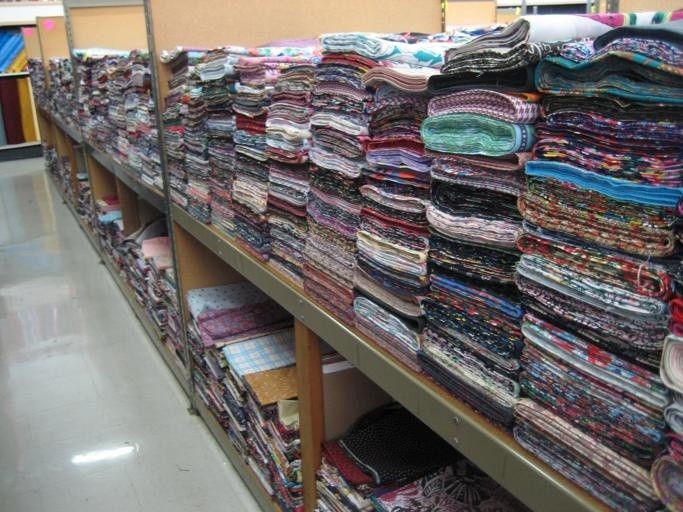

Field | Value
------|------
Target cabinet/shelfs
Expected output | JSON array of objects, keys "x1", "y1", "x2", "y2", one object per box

[
  {"x1": 0, "y1": 20, "x2": 42, "y2": 147},
  {"x1": 24, "y1": 0, "x2": 683, "y2": 512}
]
